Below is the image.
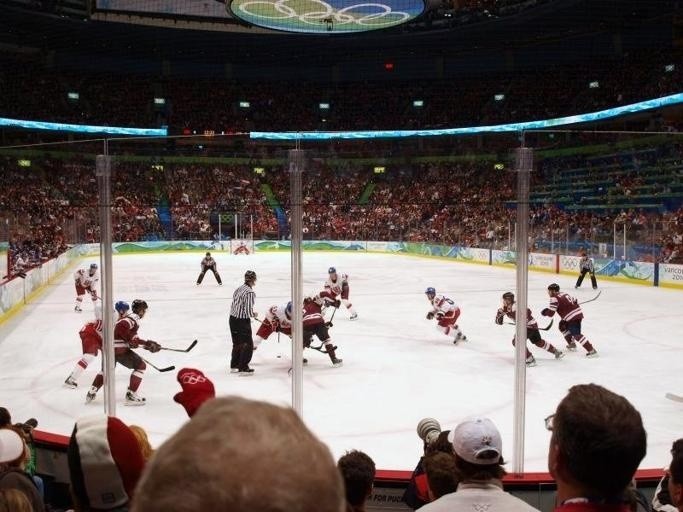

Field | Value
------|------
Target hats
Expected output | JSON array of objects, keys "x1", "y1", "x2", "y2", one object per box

[
  {"x1": 0, "y1": 429, "x2": 23, "y2": 463},
  {"x1": 66, "y1": 414, "x2": 145, "y2": 511},
  {"x1": 447, "y1": 414, "x2": 503, "y2": 466}
]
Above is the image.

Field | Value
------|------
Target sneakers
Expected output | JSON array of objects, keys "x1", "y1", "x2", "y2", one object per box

[
  {"x1": 526, "y1": 356, "x2": 535, "y2": 364},
  {"x1": 238, "y1": 365, "x2": 254, "y2": 372},
  {"x1": 74, "y1": 306, "x2": 82, "y2": 311},
  {"x1": 453, "y1": 332, "x2": 462, "y2": 344},
  {"x1": 64, "y1": 377, "x2": 78, "y2": 387},
  {"x1": 85, "y1": 391, "x2": 96, "y2": 402},
  {"x1": 566, "y1": 344, "x2": 575, "y2": 349},
  {"x1": 585, "y1": 349, "x2": 597, "y2": 356},
  {"x1": 350, "y1": 312, "x2": 357, "y2": 319},
  {"x1": 126, "y1": 389, "x2": 146, "y2": 402},
  {"x1": 231, "y1": 361, "x2": 239, "y2": 368},
  {"x1": 332, "y1": 358, "x2": 342, "y2": 364}
]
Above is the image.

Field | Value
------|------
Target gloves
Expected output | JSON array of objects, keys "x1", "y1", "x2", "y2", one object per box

[
  {"x1": 436, "y1": 313, "x2": 443, "y2": 321},
  {"x1": 272, "y1": 320, "x2": 280, "y2": 332},
  {"x1": 426, "y1": 313, "x2": 434, "y2": 320},
  {"x1": 91, "y1": 294, "x2": 97, "y2": 301},
  {"x1": 173, "y1": 367, "x2": 215, "y2": 417},
  {"x1": 495, "y1": 316, "x2": 503, "y2": 325},
  {"x1": 541, "y1": 308, "x2": 549, "y2": 316},
  {"x1": 145, "y1": 340, "x2": 161, "y2": 353},
  {"x1": 75, "y1": 279, "x2": 81, "y2": 287},
  {"x1": 325, "y1": 321, "x2": 334, "y2": 329},
  {"x1": 128, "y1": 337, "x2": 140, "y2": 349},
  {"x1": 333, "y1": 299, "x2": 342, "y2": 309}
]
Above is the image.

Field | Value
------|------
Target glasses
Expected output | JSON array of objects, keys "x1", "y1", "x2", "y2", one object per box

[
  {"x1": 663, "y1": 469, "x2": 673, "y2": 479},
  {"x1": 544, "y1": 414, "x2": 557, "y2": 430}
]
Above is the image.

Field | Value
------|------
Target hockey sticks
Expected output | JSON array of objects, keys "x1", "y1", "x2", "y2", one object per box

[
  {"x1": 327, "y1": 306, "x2": 340, "y2": 325},
  {"x1": 573, "y1": 290, "x2": 602, "y2": 305},
  {"x1": 254, "y1": 315, "x2": 337, "y2": 354},
  {"x1": 136, "y1": 340, "x2": 203, "y2": 353},
  {"x1": 505, "y1": 318, "x2": 554, "y2": 331},
  {"x1": 142, "y1": 357, "x2": 177, "y2": 373},
  {"x1": 311, "y1": 306, "x2": 339, "y2": 350}
]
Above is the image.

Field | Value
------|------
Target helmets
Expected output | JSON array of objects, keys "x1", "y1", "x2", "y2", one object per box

[
  {"x1": 547, "y1": 283, "x2": 559, "y2": 290},
  {"x1": 502, "y1": 292, "x2": 513, "y2": 299},
  {"x1": 328, "y1": 266, "x2": 337, "y2": 275},
  {"x1": 303, "y1": 296, "x2": 313, "y2": 307},
  {"x1": 114, "y1": 301, "x2": 129, "y2": 311},
  {"x1": 244, "y1": 270, "x2": 257, "y2": 282},
  {"x1": 425, "y1": 288, "x2": 435, "y2": 294},
  {"x1": 286, "y1": 302, "x2": 292, "y2": 313},
  {"x1": 90, "y1": 264, "x2": 98, "y2": 269},
  {"x1": 131, "y1": 299, "x2": 148, "y2": 311}
]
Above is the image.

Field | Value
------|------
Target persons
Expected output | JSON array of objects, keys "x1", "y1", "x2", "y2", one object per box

[
  {"x1": 539, "y1": 282, "x2": 597, "y2": 356},
  {"x1": 494, "y1": 291, "x2": 557, "y2": 364},
  {"x1": 574, "y1": 252, "x2": 598, "y2": 288},
  {"x1": 62, "y1": 302, "x2": 133, "y2": 387},
  {"x1": 0, "y1": 366, "x2": 682, "y2": 511},
  {"x1": 228, "y1": 270, "x2": 258, "y2": 372},
  {"x1": 424, "y1": 285, "x2": 466, "y2": 345},
  {"x1": 251, "y1": 265, "x2": 358, "y2": 365},
  {"x1": 71, "y1": 254, "x2": 99, "y2": 313},
  {"x1": 195, "y1": 253, "x2": 221, "y2": 285},
  {"x1": 0, "y1": 118, "x2": 680, "y2": 281},
  {"x1": 86, "y1": 298, "x2": 161, "y2": 406}
]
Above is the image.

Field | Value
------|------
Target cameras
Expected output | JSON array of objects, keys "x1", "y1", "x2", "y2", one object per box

[
  {"x1": 16, "y1": 415, "x2": 39, "y2": 434},
  {"x1": 416, "y1": 416, "x2": 442, "y2": 447}
]
